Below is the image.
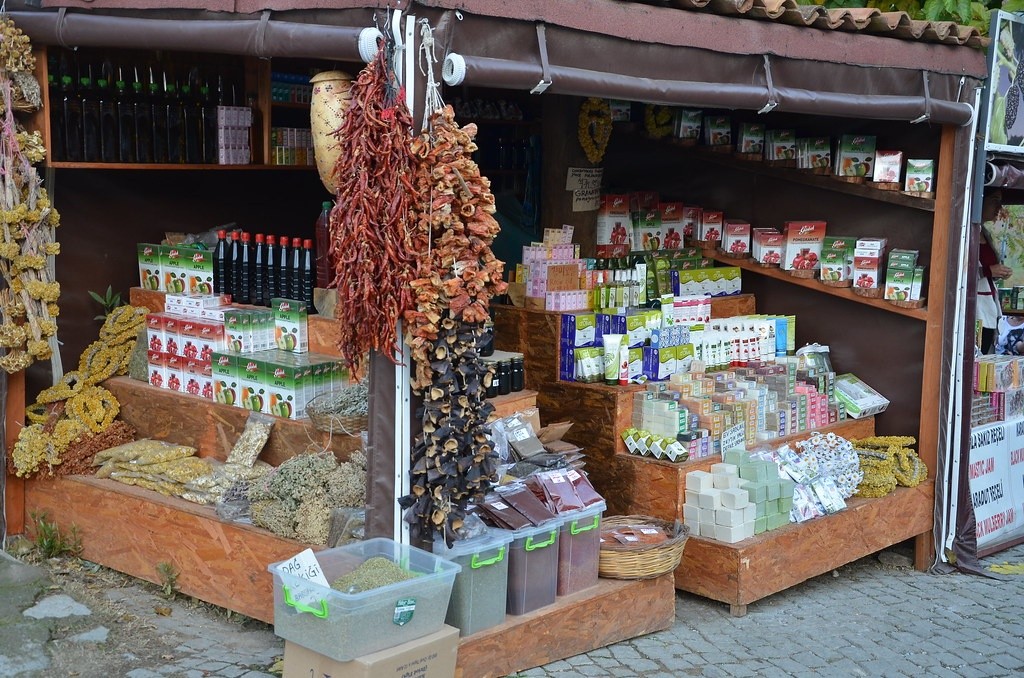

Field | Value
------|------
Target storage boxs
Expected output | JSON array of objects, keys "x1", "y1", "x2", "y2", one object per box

[
  {"x1": 506, "y1": 517, "x2": 563, "y2": 615},
  {"x1": 268, "y1": 538, "x2": 461, "y2": 661},
  {"x1": 974, "y1": 353, "x2": 1023, "y2": 423},
  {"x1": 282, "y1": 624, "x2": 461, "y2": 678},
  {"x1": 999, "y1": 284, "x2": 1024, "y2": 310},
  {"x1": 555, "y1": 500, "x2": 607, "y2": 596},
  {"x1": 515, "y1": 110, "x2": 932, "y2": 524},
  {"x1": 433, "y1": 525, "x2": 514, "y2": 637},
  {"x1": 138, "y1": 241, "x2": 349, "y2": 423}
]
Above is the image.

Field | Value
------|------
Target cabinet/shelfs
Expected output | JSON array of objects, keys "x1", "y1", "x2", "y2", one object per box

[
  {"x1": 28, "y1": 45, "x2": 315, "y2": 170},
  {"x1": 24, "y1": 286, "x2": 674, "y2": 678},
  {"x1": 487, "y1": 293, "x2": 934, "y2": 618},
  {"x1": 594, "y1": 100, "x2": 956, "y2": 322}
]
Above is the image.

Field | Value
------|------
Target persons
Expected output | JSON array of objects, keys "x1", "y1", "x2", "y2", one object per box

[
  {"x1": 975, "y1": 186, "x2": 1013, "y2": 358},
  {"x1": 998, "y1": 315, "x2": 1024, "y2": 356}
]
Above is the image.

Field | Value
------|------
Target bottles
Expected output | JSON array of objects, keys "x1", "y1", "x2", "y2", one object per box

[
  {"x1": 473, "y1": 134, "x2": 533, "y2": 172},
  {"x1": 246, "y1": 93, "x2": 255, "y2": 124},
  {"x1": 271, "y1": 72, "x2": 314, "y2": 104},
  {"x1": 315, "y1": 202, "x2": 336, "y2": 288},
  {"x1": 593, "y1": 268, "x2": 639, "y2": 314},
  {"x1": 213, "y1": 230, "x2": 315, "y2": 315},
  {"x1": 631, "y1": 251, "x2": 679, "y2": 307},
  {"x1": 47, "y1": 59, "x2": 225, "y2": 165},
  {"x1": 482, "y1": 355, "x2": 524, "y2": 398}
]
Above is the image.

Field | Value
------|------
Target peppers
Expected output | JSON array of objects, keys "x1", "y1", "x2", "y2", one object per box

[{"x1": 323, "y1": 48, "x2": 424, "y2": 383}]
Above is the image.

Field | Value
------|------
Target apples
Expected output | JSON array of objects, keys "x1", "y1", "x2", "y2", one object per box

[
  {"x1": 644, "y1": 128, "x2": 926, "y2": 301},
  {"x1": 144, "y1": 276, "x2": 294, "y2": 418}
]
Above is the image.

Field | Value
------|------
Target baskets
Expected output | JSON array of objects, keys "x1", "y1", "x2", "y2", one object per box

[
  {"x1": 305, "y1": 406, "x2": 368, "y2": 434},
  {"x1": 598, "y1": 515, "x2": 690, "y2": 580}
]
